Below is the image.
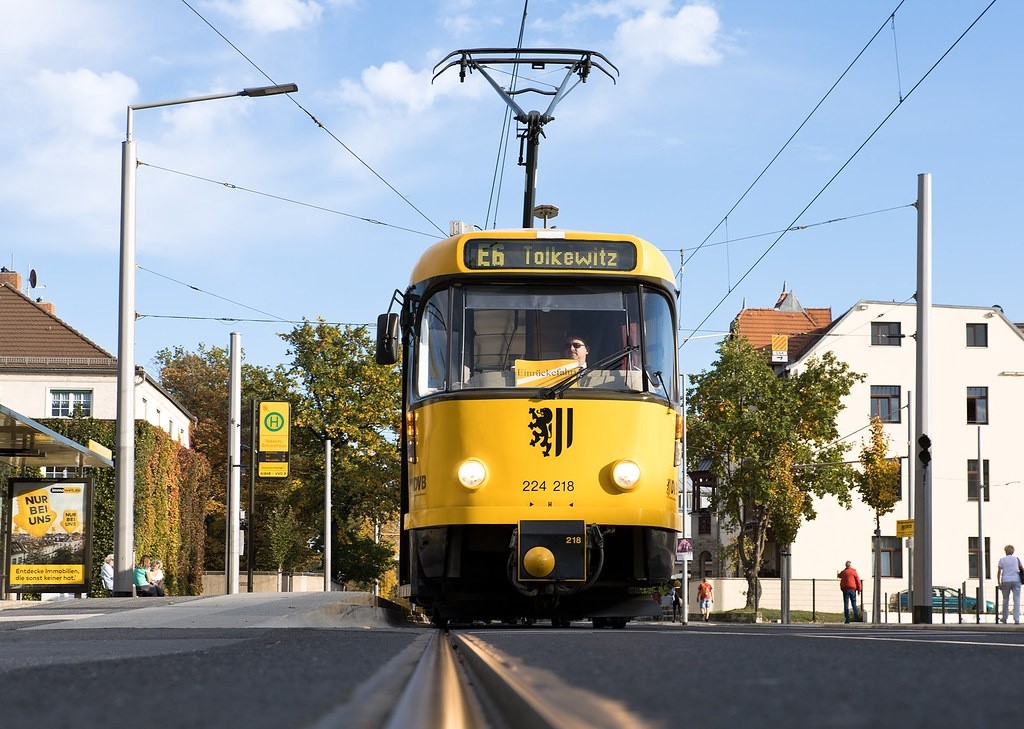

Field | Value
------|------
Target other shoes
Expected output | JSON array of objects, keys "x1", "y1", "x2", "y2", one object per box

[
  {"x1": 1000, "y1": 618, "x2": 1006, "y2": 624},
  {"x1": 1015, "y1": 621, "x2": 1019, "y2": 624}
]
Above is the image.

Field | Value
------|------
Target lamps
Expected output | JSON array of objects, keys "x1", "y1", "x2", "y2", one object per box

[
  {"x1": 989, "y1": 312, "x2": 996, "y2": 318},
  {"x1": 861, "y1": 304, "x2": 868, "y2": 310}
]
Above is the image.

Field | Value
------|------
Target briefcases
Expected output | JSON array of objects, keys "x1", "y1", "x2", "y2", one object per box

[{"x1": 1018, "y1": 568, "x2": 1024, "y2": 585}]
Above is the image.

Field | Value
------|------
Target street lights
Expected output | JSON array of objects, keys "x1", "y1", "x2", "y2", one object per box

[{"x1": 112, "y1": 81, "x2": 300, "y2": 598}]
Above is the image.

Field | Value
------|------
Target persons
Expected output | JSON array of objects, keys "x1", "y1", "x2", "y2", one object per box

[
  {"x1": 562, "y1": 330, "x2": 591, "y2": 369},
  {"x1": 101, "y1": 554, "x2": 165, "y2": 597},
  {"x1": 998, "y1": 545, "x2": 1023, "y2": 624},
  {"x1": 697, "y1": 576, "x2": 713, "y2": 622},
  {"x1": 838, "y1": 561, "x2": 861, "y2": 623},
  {"x1": 672, "y1": 580, "x2": 683, "y2": 623}
]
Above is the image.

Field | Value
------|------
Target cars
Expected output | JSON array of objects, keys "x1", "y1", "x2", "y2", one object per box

[{"x1": 889, "y1": 584, "x2": 995, "y2": 614}]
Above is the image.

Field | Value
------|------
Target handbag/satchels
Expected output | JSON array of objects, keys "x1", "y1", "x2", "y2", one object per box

[{"x1": 679, "y1": 598, "x2": 683, "y2": 607}]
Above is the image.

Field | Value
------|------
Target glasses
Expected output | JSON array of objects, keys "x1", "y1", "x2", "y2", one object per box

[{"x1": 565, "y1": 342, "x2": 587, "y2": 348}]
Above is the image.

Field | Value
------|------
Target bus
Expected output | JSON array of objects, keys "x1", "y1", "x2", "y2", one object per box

[{"x1": 376, "y1": 228, "x2": 684, "y2": 626}]
